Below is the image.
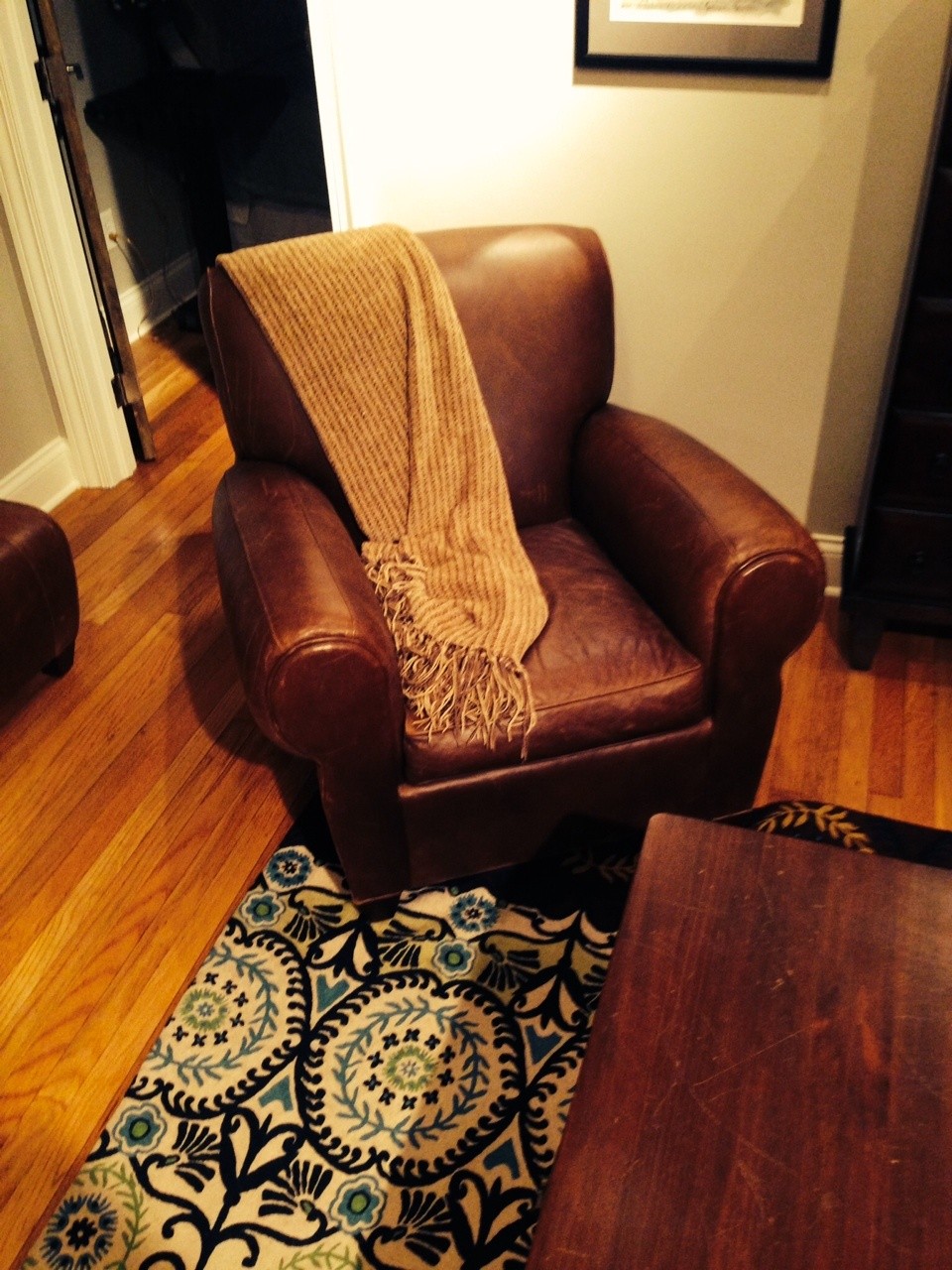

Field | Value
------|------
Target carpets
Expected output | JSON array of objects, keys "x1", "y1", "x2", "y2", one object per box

[{"x1": 4, "y1": 788, "x2": 952, "y2": 1269}]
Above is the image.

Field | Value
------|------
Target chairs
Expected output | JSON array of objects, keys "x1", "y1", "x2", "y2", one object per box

[
  {"x1": 0, "y1": 500, "x2": 79, "y2": 693},
  {"x1": 197, "y1": 224, "x2": 828, "y2": 923}
]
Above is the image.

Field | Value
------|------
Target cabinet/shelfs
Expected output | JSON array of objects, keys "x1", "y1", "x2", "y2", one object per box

[{"x1": 840, "y1": 19, "x2": 952, "y2": 670}]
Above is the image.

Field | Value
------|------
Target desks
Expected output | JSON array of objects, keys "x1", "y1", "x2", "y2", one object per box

[{"x1": 529, "y1": 815, "x2": 952, "y2": 1270}]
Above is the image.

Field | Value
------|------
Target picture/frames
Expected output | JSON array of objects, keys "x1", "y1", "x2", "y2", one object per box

[{"x1": 574, "y1": 1, "x2": 841, "y2": 77}]
